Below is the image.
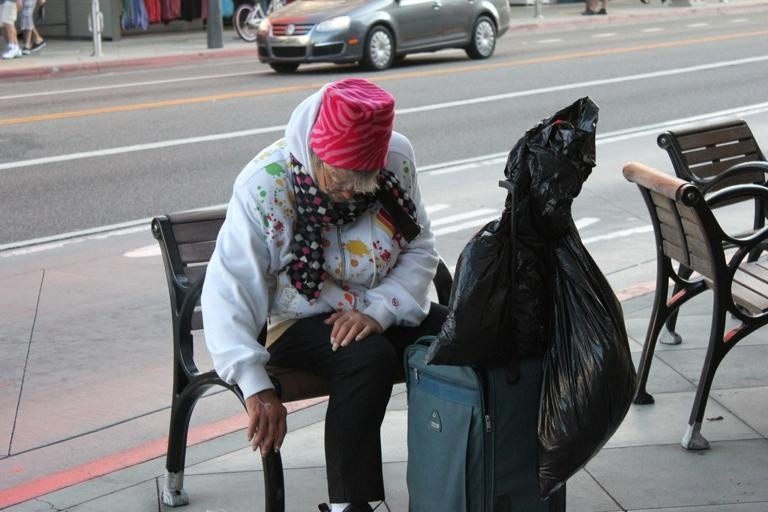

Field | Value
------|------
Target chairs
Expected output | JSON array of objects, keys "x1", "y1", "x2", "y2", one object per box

[
  {"x1": 147, "y1": 198, "x2": 457, "y2": 511},
  {"x1": 617, "y1": 161, "x2": 767, "y2": 455},
  {"x1": 651, "y1": 111, "x2": 768, "y2": 346}
]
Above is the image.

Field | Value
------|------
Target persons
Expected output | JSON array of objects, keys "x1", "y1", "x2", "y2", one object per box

[
  {"x1": 583, "y1": 0, "x2": 607, "y2": 15},
  {"x1": 201, "y1": 79, "x2": 448, "y2": 512},
  {"x1": 20, "y1": 0, "x2": 47, "y2": 54},
  {"x1": 0, "y1": 0, "x2": 22, "y2": 59}
]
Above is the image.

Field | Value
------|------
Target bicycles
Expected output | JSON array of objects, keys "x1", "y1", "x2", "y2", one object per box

[{"x1": 232, "y1": 0, "x2": 284, "y2": 43}]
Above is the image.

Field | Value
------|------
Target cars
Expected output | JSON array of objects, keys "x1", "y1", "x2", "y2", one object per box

[{"x1": 255, "y1": 0, "x2": 511, "y2": 76}]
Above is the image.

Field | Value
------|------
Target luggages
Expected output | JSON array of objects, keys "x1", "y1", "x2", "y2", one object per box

[{"x1": 401, "y1": 333, "x2": 567, "y2": 512}]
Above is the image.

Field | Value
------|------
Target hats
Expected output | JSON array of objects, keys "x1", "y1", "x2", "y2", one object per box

[{"x1": 307, "y1": 78, "x2": 396, "y2": 172}]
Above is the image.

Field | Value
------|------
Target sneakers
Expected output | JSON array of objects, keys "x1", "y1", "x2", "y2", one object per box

[
  {"x1": 21, "y1": 40, "x2": 47, "y2": 55},
  {"x1": 0, "y1": 43, "x2": 22, "y2": 59}
]
